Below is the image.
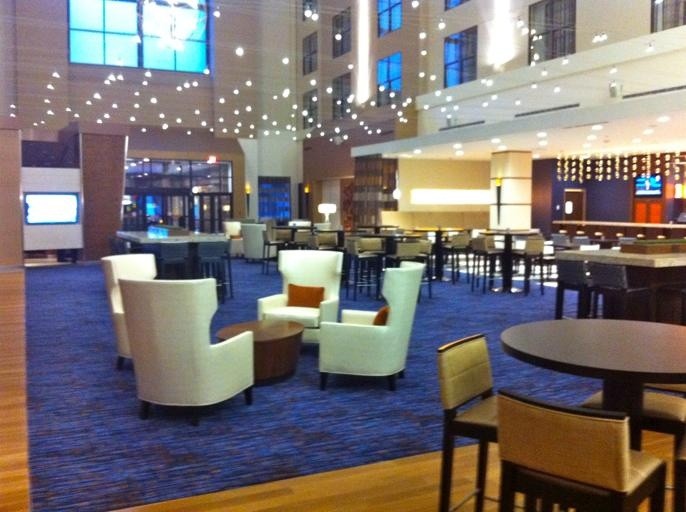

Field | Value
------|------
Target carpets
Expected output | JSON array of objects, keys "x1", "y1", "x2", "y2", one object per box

[{"x1": 23, "y1": 256, "x2": 686, "y2": 510}]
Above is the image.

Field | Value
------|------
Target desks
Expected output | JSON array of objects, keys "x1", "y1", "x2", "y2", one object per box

[
  {"x1": 555, "y1": 247, "x2": 685, "y2": 324},
  {"x1": 500, "y1": 319, "x2": 686, "y2": 453},
  {"x1": 110, "y1": 229, "x2": 233, "y2": 304},
  {"x1": 215, "y1": 318, "x2": 305, "y2": 387}
]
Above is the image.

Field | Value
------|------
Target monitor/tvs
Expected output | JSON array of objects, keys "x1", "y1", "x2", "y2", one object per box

[
  {"x1": 634, "y1": 174, "x2": 663, "y2": 197},
  {"x1": 23, "y1": 192, "x2": 80, "y2": 225}
]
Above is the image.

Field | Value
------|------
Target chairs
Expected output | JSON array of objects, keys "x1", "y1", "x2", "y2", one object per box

[
  {"x1": 436, "y1": 334, "x2": 536, "y2": 512},
  {"x1": 588, "y1": 261, "x2": 655, "y2": 321},
  {"x1": 494, "y1": 387, "x2": 667, "y2": 512},
  {"x1": 225, "y1": 220, "x2": 686, "y2": 302},
  {"x1": 555, "y1": 259, "x2": 601, "y2": 320},
  {"x1": 651, "y1": 280, "x2": 685, "y2": 325},
  {"x1": 103, "y1": 253, "x2": 157, "y2": 372},
  {"x1": 577, "y1": 376, "x2": 686, "y2": 512},
  {"x1": 319, "y1": 259, "x2": 427, "y2": 391},
  {"x1": 256, "y1": 249, "x2": 344, "y2": 346},
  {"x1": 116, "y1": 277, "x2": 254, "y2": 425}
]
